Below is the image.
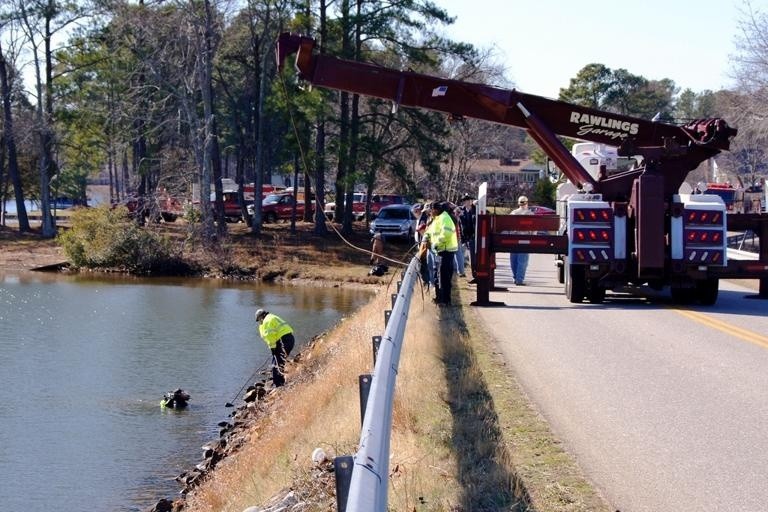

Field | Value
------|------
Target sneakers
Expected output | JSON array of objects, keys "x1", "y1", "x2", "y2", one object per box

[{"x1": 513, "y1": 278, "x2": 526, "y2": 286}]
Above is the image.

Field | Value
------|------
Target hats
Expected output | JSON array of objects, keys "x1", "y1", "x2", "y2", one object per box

[
  {"x1": 420, "y1": 203, "x2": 431, "y2": 213},
  {"x1": 517, "y1": 195, "x2": 529, "y2": 206},
  {"x1": 459, "y1": 195, "x2": 477, "y2": 202},
  {"x1": 255, "y1": 309, "x2": 266, "y2": 322}
]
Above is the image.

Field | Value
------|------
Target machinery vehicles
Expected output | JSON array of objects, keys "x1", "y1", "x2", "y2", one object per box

[{"x1": 274, "y1": 32, "x2": 767, "y2": 309}]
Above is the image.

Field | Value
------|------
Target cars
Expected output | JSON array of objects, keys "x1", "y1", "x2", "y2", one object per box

[
  {"x1": 370, "y1": 204, "x2": 418, "y2": 244},
  {"x1": 113, "y1": 178, "x2": 408, "y2": 223}
]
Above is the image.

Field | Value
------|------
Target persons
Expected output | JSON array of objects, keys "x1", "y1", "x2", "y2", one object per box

[
  {"x1": 369, "y1": 229, "x2": 387, "y2": 267},
  {"x1": 255, "y1": 309, "x2": 294, "y2": 388},
  {"x1": 507, "y1": 196, "x2": 537, "y2": 286},
  {"x1": 411, "y1": 196, "x2": 476, "y2": 305},
  {"x1": 160, "y1": 388, "x2": 190, "y2": 412}
]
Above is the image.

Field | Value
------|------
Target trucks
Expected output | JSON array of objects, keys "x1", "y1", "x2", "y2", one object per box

[{"x1": 691, "y1": 178, "x2": 735, "y2": 211}]
